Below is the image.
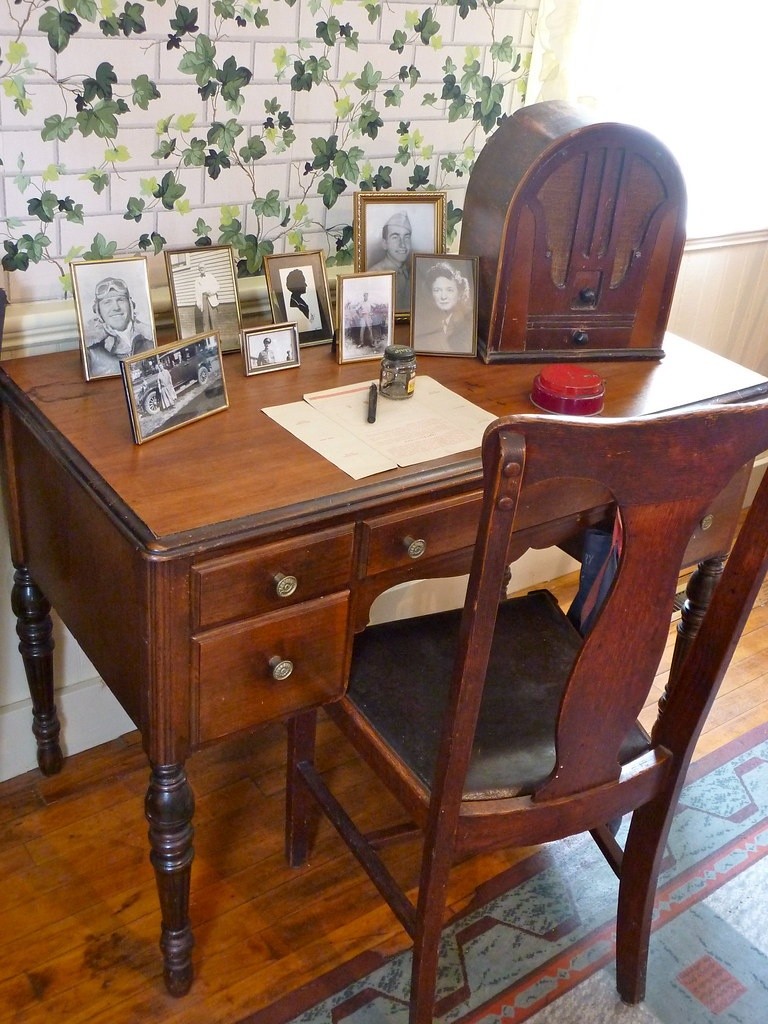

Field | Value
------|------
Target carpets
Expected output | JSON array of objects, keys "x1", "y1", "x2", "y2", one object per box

[{"x1": 241, "y1": 721, "x2": 768, "y2": 1024}]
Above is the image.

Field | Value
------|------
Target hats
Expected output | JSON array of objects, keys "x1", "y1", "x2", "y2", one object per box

[
  {"x1": 93, "y1": 277, "x2": 135, "y2": 322},
  {"x1": 384, "y1": 213, "x2": 412, "y2": 234},
  {"x1": 263, "y1": 338, "x2": 271, "y2": 343}
]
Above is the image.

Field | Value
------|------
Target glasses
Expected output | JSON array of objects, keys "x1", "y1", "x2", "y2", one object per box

[{"x1": 96, "y1": 279, "x2": 130, "y2": 302}]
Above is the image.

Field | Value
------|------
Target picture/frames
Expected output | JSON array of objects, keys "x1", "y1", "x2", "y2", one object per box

[
  {"x1": 237, "y1": 322, "x2": 302, "y2": 377},
  {"x1": 336, "y1": 269, "x2": 396, "y2": 364},
  {"x1": 408, "y1": 252, "x2": 479, "y2": 360},
  {"x1": 164, "y1": 243, "x2": 244, "y2": 355},
  {"x1": 263, "y1": 249, "x2": 333, "y2": 349},
  {"x1": 354, "y1": 191, "x2": 447, "y2": 323},
  {"x1": 118, "y1": 329, "x2": 229, "y2": 443},
  {"x1": 70, "y1": 256, "x2": 159, "y2": 381}
]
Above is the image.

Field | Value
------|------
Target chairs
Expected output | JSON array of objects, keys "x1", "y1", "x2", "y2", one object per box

[{"x1": 292, "y1": 398, "x2": 768, "y2": 1024}]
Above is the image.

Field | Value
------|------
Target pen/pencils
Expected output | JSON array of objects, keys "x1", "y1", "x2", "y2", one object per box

[{"x1": 368, "y1": 382, "x2": 377, "y2": 425}]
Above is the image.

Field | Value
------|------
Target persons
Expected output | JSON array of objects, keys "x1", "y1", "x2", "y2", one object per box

[{"x1": 85, "y1": 213, "x2": 474, "y2": 411}]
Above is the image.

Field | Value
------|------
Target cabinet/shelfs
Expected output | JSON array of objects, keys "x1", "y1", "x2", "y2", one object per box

[{"x1": 0, "y1": 321, "x2": 768, "y2": 998}]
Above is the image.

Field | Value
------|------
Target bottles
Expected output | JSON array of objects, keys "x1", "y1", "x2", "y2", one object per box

[{"x1": 379, "y1": 344, "x2": 418, "y2": 399}]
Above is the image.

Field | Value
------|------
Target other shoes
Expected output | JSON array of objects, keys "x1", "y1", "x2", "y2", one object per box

[
  {"x1": 371, "y1": 344, "x2": 375, "y2": 347},
  {"x1": 357, "y1": 344, "x2": 363, "y2": 347}
]
